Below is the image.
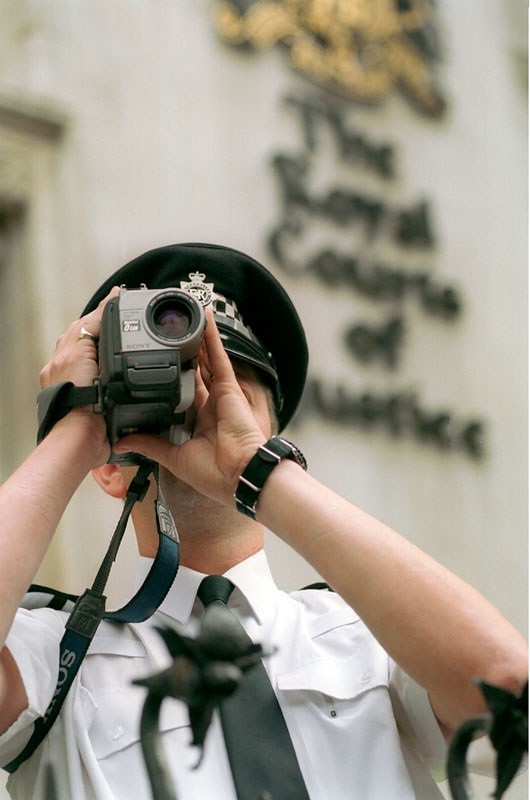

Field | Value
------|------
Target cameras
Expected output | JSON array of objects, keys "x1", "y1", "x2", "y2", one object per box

[{"x1": 96, "y1": 287, "x2": 204, "y2": 467}]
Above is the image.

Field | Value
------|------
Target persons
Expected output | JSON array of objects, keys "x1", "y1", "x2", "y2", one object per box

[{"x1": 0, "y1": 241, "x2": 528, "y2": 800}]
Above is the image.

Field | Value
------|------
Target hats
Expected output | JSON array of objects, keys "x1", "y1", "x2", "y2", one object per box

[{"x1": 81, "y1": 242, "x2": 310, "y2": 436}]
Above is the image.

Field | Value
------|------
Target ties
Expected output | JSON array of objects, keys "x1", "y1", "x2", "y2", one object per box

[{"x1": 198, "y1": 575, "x2": 312, "y2": 800}]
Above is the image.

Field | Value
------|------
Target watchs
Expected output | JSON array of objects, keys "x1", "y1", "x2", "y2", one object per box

[{"x1": 233, "y1": 436, "x2": 309, "y2": 520}]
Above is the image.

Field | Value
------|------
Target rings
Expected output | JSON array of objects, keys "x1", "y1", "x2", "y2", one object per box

[{"x1": 78, "y1": 327, "x2": 97, "y2": 340}]
